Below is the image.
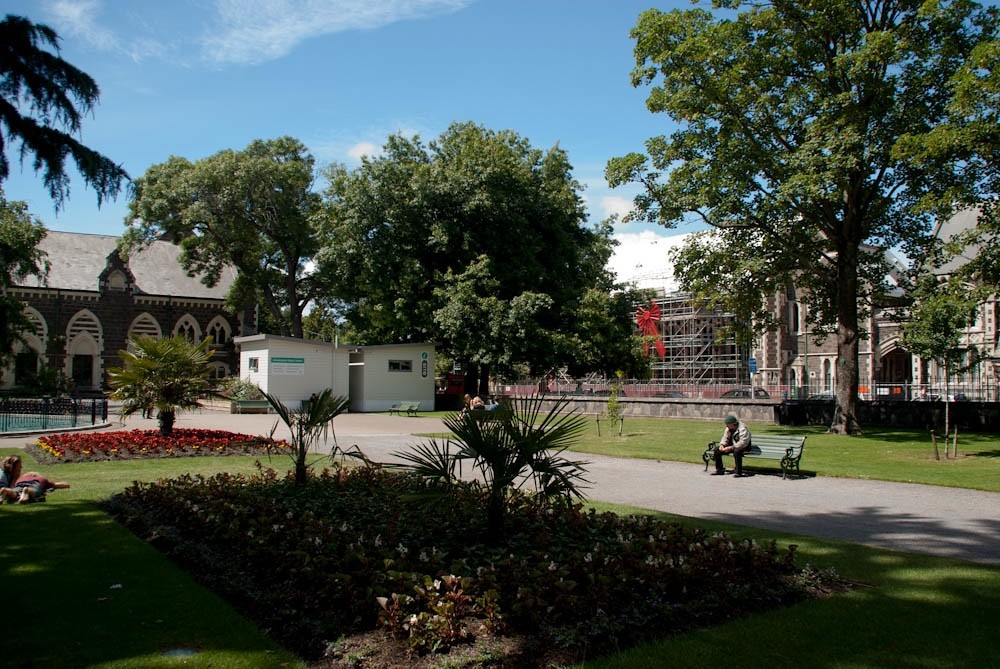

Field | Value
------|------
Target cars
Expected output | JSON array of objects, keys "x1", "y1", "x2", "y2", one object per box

[
  {"x1": 909, "y1": 392, "x2": 968, "y2": 402},
  {"x1": 807, "y1": 394, "x2": 837, "y2": 401},
  {"x1": 594, "y1": 388, "x2": 627, "y2": 397},
  {"x1": 720, "y1": 386, "x2": 770, "y2": 399},
  {"x1": 643, "y1": 390, "x2": 687, "y2": 398}
]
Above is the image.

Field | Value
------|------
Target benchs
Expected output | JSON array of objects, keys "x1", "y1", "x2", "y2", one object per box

[
  {"x1": 236, "y1": 400, "x2": 273, "y2": 414},
  {"x1": 389, "y1": 400, "x2": 420, "y2": 416},
  {"x1": 470, "y1": 409, "x2": 514, "y2": 431},
  {"x1": 71, "y1": 389, "x2": 109, "y2": 404},
  {"x1": 703, "y1": 435, "x2": 807, "y2": 480}
]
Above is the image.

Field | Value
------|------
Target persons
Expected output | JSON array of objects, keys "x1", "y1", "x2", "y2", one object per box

[
  {"x1": 142, "y1": 408, "x2": 152, "y2": 419},
  {"x1": 0, "y1": 455, "x2": 70, "y2": 505},
  {"x1": 710, "y1": 415, "x2": 751, "y2": 477},
  {"x1": 454, "y1": 394, "x2": 501, "y2": 421}
]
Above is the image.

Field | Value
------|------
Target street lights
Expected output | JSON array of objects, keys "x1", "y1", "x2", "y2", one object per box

[{"x1": 799, "y1": 295, "x2": 810, "y2": 398}]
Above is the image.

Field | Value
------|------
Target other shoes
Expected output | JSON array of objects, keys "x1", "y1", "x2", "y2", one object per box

[
  {"x1": 712, "y1": 472, "x2": 724, "y2": 475},
  {"x1": 735, "y1": 472, "x2": 742, "y2": 477}
]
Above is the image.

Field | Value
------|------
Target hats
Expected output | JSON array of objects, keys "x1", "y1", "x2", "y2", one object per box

[{"x1": 725, "y1": 416, "x2": 736, "y2": 423}]
